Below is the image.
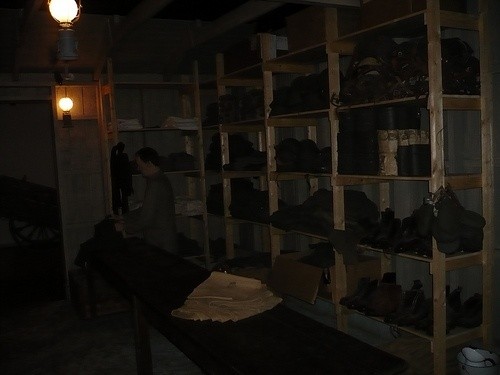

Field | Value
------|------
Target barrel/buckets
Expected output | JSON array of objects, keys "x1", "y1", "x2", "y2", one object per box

[{"x1": 457, "y1": 348, "x2": 500, "y2": 375}]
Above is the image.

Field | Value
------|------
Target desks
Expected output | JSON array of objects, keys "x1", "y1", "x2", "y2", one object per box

[{"x1": 93, "y1": 239, "x2": 411, "y2": 375}]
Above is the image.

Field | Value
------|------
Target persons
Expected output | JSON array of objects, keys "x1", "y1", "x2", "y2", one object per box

[{"x1": 109, "y1": 146, "x2": 177, "y2": 254}]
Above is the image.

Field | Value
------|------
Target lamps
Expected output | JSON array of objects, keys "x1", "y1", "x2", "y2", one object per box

[
  {"x1": 57, "y1": 97, "x2": 74, "y2": 128},
  {"x1": 46, "y1": 0, "x2": 82, "y2": 61}
]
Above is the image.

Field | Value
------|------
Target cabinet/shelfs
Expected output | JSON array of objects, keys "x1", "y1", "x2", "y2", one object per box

[{"x1": 98, "y1": 0, "x2": 495, "y2": 375}]
